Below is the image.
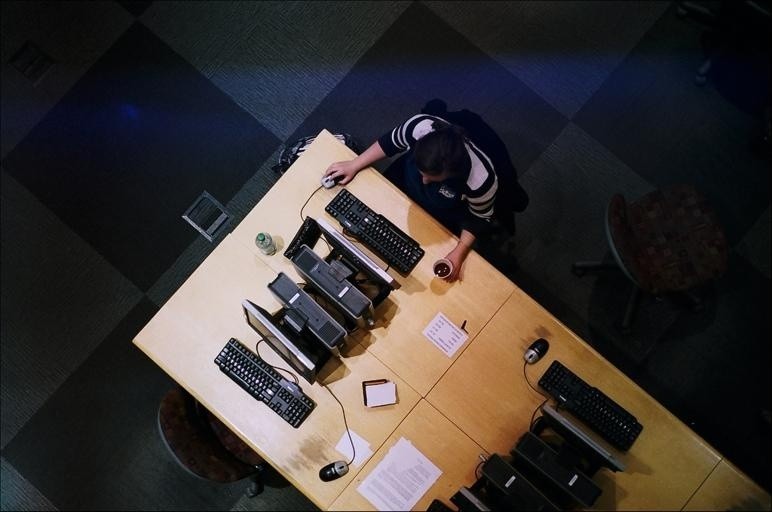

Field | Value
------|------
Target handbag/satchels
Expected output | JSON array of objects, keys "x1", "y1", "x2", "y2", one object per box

[{"x1": 279, "y1": 133, "x2": 356, "y2": 175}]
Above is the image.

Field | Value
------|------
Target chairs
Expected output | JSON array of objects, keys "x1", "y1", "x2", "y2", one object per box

[
  {"x1": 571, "y1": 189, "x2": 730, "y2": 334},
  {"x1": 157, "y1": 386, "x2": 276, "y2": 499}
]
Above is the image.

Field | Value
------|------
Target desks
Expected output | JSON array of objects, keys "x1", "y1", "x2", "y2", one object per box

[
  {"x1": 423, "y1": 287, "x2": 724, "y2": 510},
  {"x1": 230, "y1": 128, "x2": 516, "y2": 398},
  {"x1": 327, "y1": 398, "x2": 492, "y2": 510},
  {"x1": 133, "y1": 234, "x2": 423, "y2": 510},
  {"x1": 683, "y1": 457, "x2": 772, "y2": 511}
]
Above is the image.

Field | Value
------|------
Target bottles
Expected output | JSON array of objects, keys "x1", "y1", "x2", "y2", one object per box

[{"x1": 255, "y1": 231, "x2": 277, "y2": 256}]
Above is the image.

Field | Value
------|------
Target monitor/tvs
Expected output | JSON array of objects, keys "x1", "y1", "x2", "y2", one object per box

[
  {"x1": 241, "y1": 298, "x2": 333, "y2": 385},
  {"x1": 315, "y1": 217, "x2": 401, "y2": 307},
  {"x1": 535, "y1": 403, "x2": 626, "y2": 479},
  {"x1": 449, "y1": 478, "x2": 525, "y2": 512}
]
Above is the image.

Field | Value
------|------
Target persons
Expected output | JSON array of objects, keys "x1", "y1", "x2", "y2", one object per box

[{"x1": 323, "y1": 114, "x2": 500, "y2": 284}]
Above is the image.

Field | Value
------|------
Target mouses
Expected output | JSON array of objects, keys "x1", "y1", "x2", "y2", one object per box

[
  {"x1": 321, "y1": 171, "x2": 344, "y2": 189},
  {"x1": 523, "y1": 338, "x2": 549, "y2": 365},
  {"x1": 319, "y1": 461, "x2": 349, "y2": 482}
]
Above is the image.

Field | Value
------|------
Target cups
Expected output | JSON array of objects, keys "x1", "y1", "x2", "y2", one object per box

[{"x1": 431, "y1": 258, "x2": 453, "y2": 279}]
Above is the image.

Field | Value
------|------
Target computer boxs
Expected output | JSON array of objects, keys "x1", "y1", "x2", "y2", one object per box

[
  {"x1": 266, "y1": 271, "x2": 349, "y2": 357},
  {"x1": 482, "y1": 431, "x2": 604, "y2": 512},
  {"x1": 291, "y1": 244, "x2": 374, "y2": 327}
]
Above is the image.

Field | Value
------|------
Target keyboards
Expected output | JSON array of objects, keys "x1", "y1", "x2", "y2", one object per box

[
  {"x1": 213, "y1": 338, "x2": 315, "y2": 430},
  {"x1": 537, "y1": 360, "x2": 643, "y2": 454},
  {"x1": 425, "y1": 499, "x2": 454, "y2": 511},
  {"x1": 325, "y1": 188, "x2": 425, "y2": 278}
]
